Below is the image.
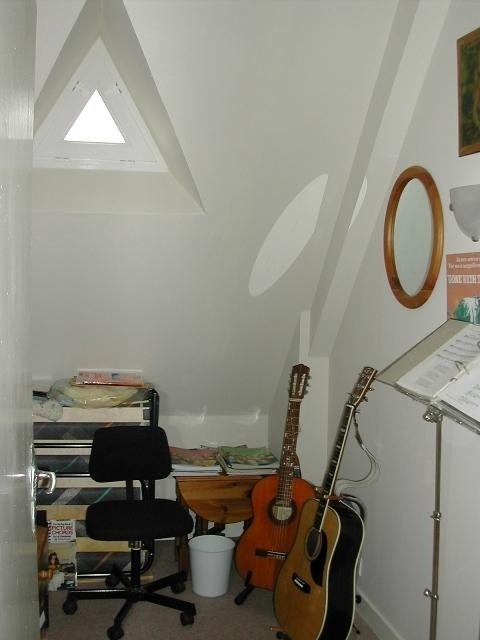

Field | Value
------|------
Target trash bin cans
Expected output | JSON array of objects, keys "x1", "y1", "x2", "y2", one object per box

[{"x1": 188, "y1": 535, "x2": 236, "y2": 598}]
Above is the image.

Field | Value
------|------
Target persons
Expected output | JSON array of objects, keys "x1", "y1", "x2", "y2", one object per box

[{"x1": 47, "y1": 552, "x2": 64, "y2": 591}]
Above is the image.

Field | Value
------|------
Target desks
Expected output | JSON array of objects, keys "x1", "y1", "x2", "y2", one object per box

[{"x1": 174, "y1": 449, "x2": 303, "y2": 581}]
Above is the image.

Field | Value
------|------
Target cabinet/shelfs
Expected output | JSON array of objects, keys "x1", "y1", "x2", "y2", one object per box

[{"x1": 31, "y1": 379, "x2": 160, "y2": 640}]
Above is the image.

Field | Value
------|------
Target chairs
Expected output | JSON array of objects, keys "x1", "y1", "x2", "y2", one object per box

[{"x1": 84, "y1": 427, "x2": 194, "y2": 640}]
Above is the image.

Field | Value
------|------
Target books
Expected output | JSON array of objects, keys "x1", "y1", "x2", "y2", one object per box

[{"x1": 164, "y1": 441, "x2": 283, "y2": 477}]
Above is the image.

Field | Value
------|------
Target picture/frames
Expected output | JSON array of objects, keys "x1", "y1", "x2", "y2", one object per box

[{"x1": 456, "y1": 28, "x2": 480, "y2": 156}]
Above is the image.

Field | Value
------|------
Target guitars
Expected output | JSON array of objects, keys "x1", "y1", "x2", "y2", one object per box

[
  {"x1": 273, "y1": 366, "x2": 366, "y2": 640},
  {"x1": 235, "y1": 363, "x2": 317, "y2": 590}
]
